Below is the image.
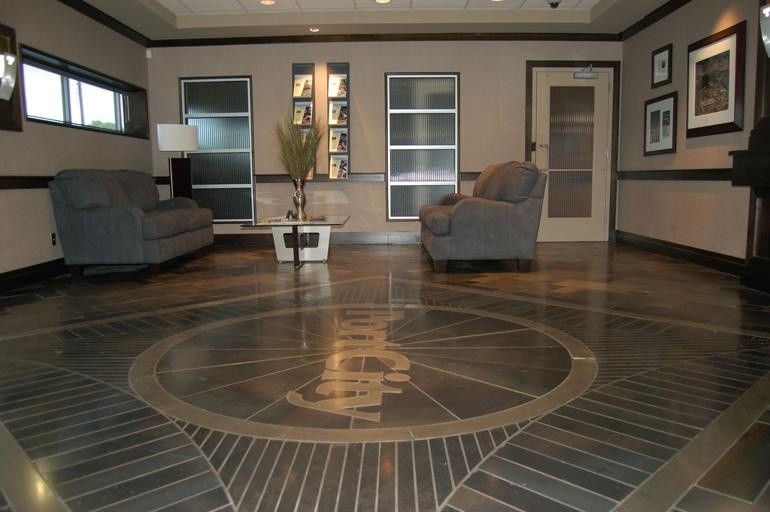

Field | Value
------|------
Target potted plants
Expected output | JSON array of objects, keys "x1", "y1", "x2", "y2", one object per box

[{"x1": 273, "y1": 118, "x2": 328, "y2": 221}]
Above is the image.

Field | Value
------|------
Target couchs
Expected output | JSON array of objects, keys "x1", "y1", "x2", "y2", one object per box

[{"x1": 49, "y1": 170, "x2": 216, "y2": 274}]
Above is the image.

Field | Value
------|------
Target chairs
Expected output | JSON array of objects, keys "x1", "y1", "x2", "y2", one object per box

[{"x1": 419, "y1": 161, "x2": 547, "y2": 272}]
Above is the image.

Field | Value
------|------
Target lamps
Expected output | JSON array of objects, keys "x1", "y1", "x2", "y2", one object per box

[{"x1": 157, "y1": 124, "x2": 200, "y2": 196}]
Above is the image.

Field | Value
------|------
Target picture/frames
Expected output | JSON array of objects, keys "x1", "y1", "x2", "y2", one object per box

[
  {"x1": 643, "y1": 19, "x2": 747, "y2": 156},
  {"x1": 0, "y1": 24, "x2": 20, "y2": 131}
]
